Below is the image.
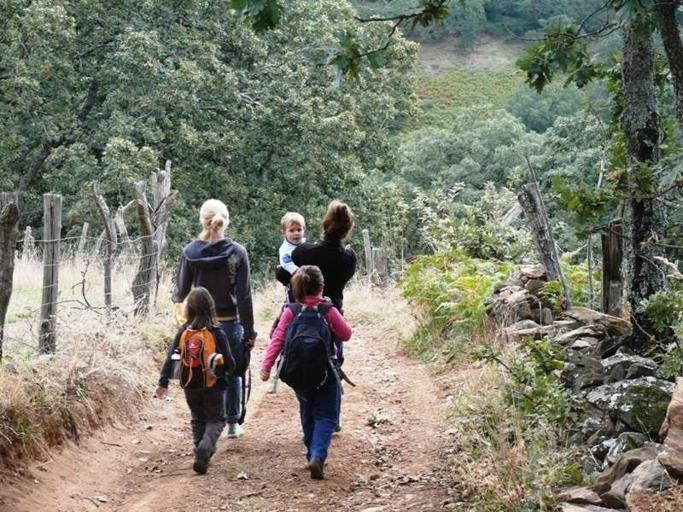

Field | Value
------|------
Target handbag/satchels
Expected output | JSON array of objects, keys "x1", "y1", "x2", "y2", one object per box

[{"x1": 235, "y1": 341, "x2": 250, "y2": 377}]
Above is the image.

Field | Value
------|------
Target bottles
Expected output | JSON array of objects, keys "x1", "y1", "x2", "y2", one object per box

[
  {"x1": 232, "y1": 322, "x2": 245, "y2": 341},
  {"x1": 169, "y1": 349, "x2": 181, "y2": 380}
]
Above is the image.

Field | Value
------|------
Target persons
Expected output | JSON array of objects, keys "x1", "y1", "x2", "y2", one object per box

[
  {"x1": 154, "y1": 287, "x2": 237, "y2": 476},
  {"x1": 169, "y1": 198, "x2": 256, "y2": 439},
  {"x1": 275, "y1": 198, "x2": 356, "y2": 432},
  {"x1": 257, "y1": 263, "x2": 353, "y2": 480},
  {"x1": 278, "y1": 211, "x2": 307, "y2": 275}
]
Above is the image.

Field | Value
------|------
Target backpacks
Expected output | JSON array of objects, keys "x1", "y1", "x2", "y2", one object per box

[
  {"x1": 275, "y1": 302, "x2": 334, "y2": 394},
  {"x1": 170, "y1": 321, "x2": 224, "y2": 390}
]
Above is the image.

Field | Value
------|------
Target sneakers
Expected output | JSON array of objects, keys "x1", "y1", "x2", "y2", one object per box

[
  {"x1": 306, "y1": 453, "x2": 325, "y2": 480},
  {"x1": 228, "y1": 425, "x2": 243, "y2": 438},
  {"x1": 193, "y1": 441, "x2": 216, "y2": 473}
]
